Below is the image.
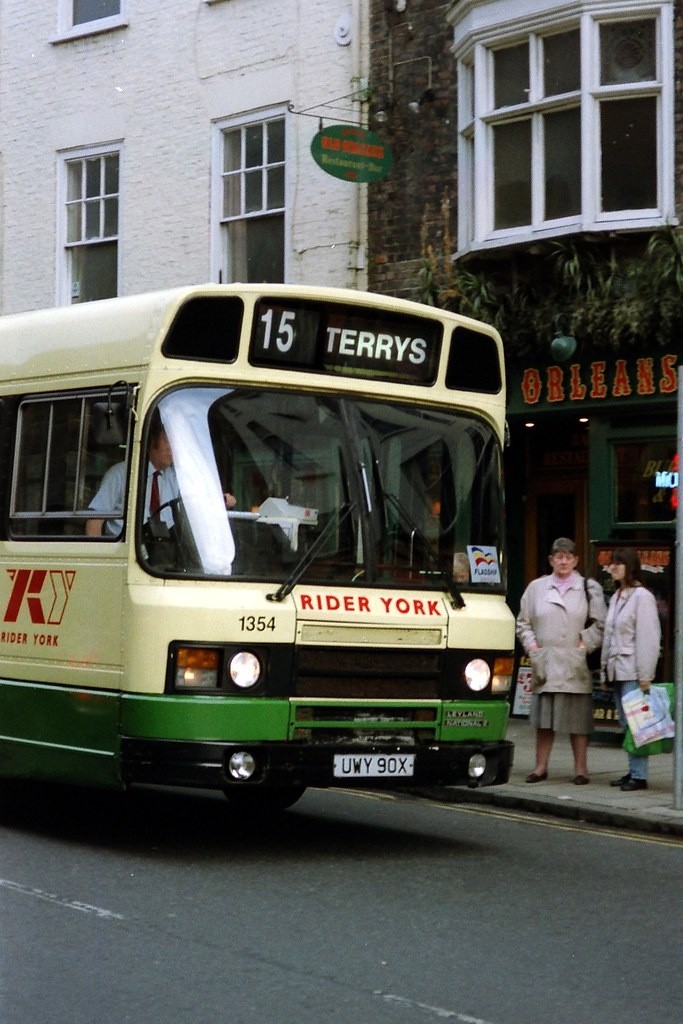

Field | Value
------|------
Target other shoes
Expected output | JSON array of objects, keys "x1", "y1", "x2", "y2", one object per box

[
  {"x1": 574, "y1": 775, "x2": 590, "y2": 785},
  {"x1": 611, "y1": 774, "x2": 632, "y2": 787},
  {"x1": 621, "y1": 778, "x2": 648, "y2": 790},
  {"x1": 526, "y1": 772, "x2": 548, "y2": 783}
]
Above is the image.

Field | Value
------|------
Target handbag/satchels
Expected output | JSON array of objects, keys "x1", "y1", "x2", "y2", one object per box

[
  {"x1": 624, "y1": 682, "x2": 675, "y2": 756},
  {"x1": 621, "y1": 686, "x2": 675, "y2": 748},
  {"x1": 584, "y1": 577, "x2": 609, "y2": 671}
]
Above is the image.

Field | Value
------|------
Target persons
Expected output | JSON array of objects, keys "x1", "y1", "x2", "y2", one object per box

[
  {"x1": 85, "y1": 419, "x2": 236, "y2": 580},
  {"x1": 517, "y1": 537, "x2": 608, "y2": 786},
  {"x1": 452, "y1": 552, "x2": 471, "y2": 584},
  {"x1": 599, "y1": 546, "x2": 661, "y2": 793}
]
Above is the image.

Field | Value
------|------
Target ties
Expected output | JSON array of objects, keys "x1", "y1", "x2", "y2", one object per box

[{"x1": 150, "y1": 471, "x2": 163, "y2": 522}]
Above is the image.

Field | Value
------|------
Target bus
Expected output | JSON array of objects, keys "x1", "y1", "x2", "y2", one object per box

[{"x1": 0, "y1": 281, "x2": 519, "y2": 821}]
